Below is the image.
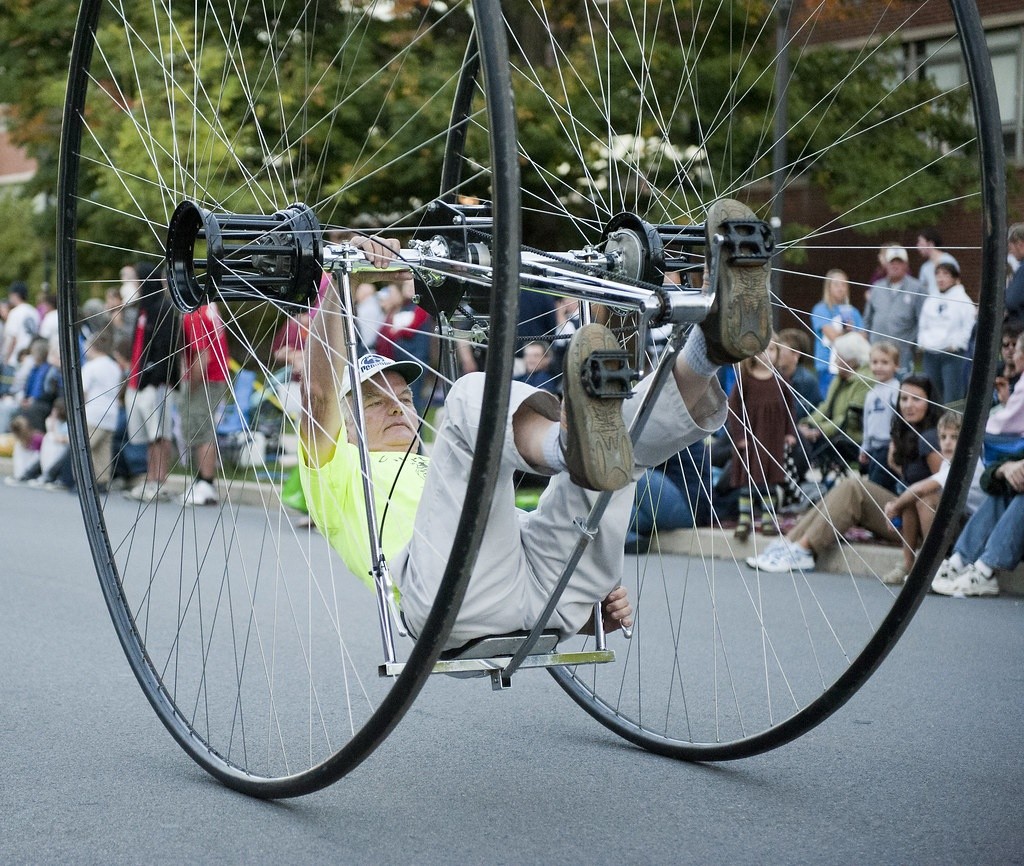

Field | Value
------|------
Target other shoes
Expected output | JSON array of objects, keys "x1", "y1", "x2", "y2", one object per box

[
  {"x1": 707, "y1": 198, "x2": 774, "y2": 367},
  {"x1": 46, "y1": 477, "x2": 72, "y2": 493},
  {"x1": 883, "y1": 563, "x2": 905, "y2": 586},
  {"x1": 562, "y1": 326, "x2": 635, "y2": 491}
]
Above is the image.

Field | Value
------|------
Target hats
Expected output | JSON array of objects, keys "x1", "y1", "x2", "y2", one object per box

[
  {"x1": 338, "y1": 350, "x2": 421, "y2": 398},
  {"x1": 886, "y1": 245, "x2": 909, "y2": 264}
]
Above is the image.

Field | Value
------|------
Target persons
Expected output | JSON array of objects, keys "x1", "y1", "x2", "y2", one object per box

[
  {"x1": 1, "y1": 224, "x2": 1024, "y2": 600},
  {"x1": 290, "y1": 199, "x2": 767, "y2": 672}
]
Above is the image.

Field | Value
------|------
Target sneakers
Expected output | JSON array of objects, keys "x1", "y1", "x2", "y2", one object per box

[
  {"x1": 929, "y1": 556, "x2": 961, "y2": 584},
  {"x1": 182, "y1": 479, "x2": 217, "y2": 508},
  {"x1": 932, "y1": 560, "x2": 999, "y2": 597},
  {"x1": 747, "y1": 535, "x2": 818, "y2": 571},
  {"x1": 132, "y1": 480, "x2": 168, "y2": 500}
]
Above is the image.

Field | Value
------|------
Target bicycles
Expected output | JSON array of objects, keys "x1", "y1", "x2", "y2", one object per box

[{"x1": 57, "y1": 1, "x2": 1010, "y2": 802}]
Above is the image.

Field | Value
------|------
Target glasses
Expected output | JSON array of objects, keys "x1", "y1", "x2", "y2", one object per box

[{"x1": 1003, "y1": 342, "x2": 1017, "y2": 347}]
